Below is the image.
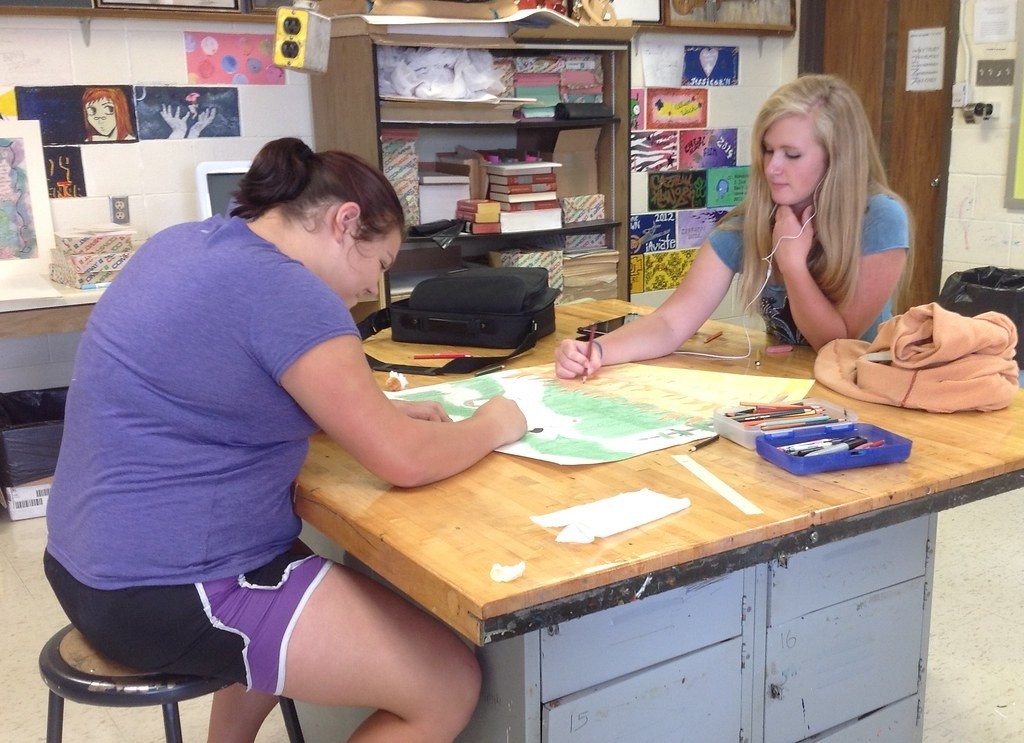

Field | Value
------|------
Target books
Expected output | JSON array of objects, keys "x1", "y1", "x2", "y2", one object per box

[
  {"x1": 418, "y1": 154, "x2": 562, "y2": 234},
  {"x1": 521, "y1": 244, "x2": 619, "y2": 301}
]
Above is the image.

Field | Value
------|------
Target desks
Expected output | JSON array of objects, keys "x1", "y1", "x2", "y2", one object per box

[{"x1": 261, "y1": 305, "x2": 1024, "y2": 743}]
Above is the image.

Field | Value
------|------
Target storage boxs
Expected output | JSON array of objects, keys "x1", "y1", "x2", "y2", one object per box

[
  {"x1": 415, "y1": 173, "x2": 471, "y2": 225},
  {"x1": 487, "y1": 247, "x2": 563, "y2": 304},
  {"x1": 538, "y1": 231, "x2": 606, "y2": 251}
]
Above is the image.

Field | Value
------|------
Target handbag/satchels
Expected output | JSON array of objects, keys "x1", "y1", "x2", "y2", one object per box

[{"x1": 357, "y1": 267, "x2": 560, "y2": 375}]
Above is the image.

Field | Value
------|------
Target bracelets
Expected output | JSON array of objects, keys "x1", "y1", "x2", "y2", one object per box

[{"x1": 593, "y1": 341, "x2": 602, "y2": 358}]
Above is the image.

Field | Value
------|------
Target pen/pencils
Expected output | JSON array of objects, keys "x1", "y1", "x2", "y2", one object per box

[
  {"x1": 724, "y1": 401, "x2": 885, "y2": 459},
  {"x1": 474, "y1": 364, "x2": 505, "y2": 377},
  {"x1": 582, "y1": 327, "x2": 594, "y2": 383},
  {"x1": 414, "y1": 353, "x2": 472, "y2": 359},
  {"x1": 689, "y1": 433, "x2": 720, "y2": 453}
]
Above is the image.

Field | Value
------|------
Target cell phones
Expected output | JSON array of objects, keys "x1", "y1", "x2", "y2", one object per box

[{"x1": 577, "y1": 313, "x2": 644, "y2": 337}]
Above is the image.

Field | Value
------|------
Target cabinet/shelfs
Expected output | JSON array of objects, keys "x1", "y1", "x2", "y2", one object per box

[{"x1": 307, "y1": 30, "x2": 630, "y2": 302}]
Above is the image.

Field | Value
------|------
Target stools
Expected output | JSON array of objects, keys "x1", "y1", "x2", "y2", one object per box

[{"x1": 37, "y1": 624, "x2": 305, "y2": 743}]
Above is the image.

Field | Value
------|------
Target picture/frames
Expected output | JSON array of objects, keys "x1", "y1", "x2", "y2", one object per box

[
  {"x1": 0, "y1": 391, "x2": 68, "y2": 521},
  {"x1": 568, "y1": 0, "x2": 797, "y2": 35}
]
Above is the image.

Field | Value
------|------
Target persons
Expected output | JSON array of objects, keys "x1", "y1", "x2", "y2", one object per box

[
  {"x1": 554, "y1": 74, "x2": 915, "y2": 379},
  {"x1": 44, "y1": 136, "x2": 528, "y2": 743}
]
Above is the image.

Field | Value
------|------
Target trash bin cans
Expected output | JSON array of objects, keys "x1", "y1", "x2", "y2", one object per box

[{"x1": 946, "y1": 265, "x2": 1024, "y2": 387}]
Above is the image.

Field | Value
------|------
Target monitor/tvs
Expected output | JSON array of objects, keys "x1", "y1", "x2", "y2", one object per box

[{"x1": 196, "y1": 160, "x2": 254, "y2": 220}]
